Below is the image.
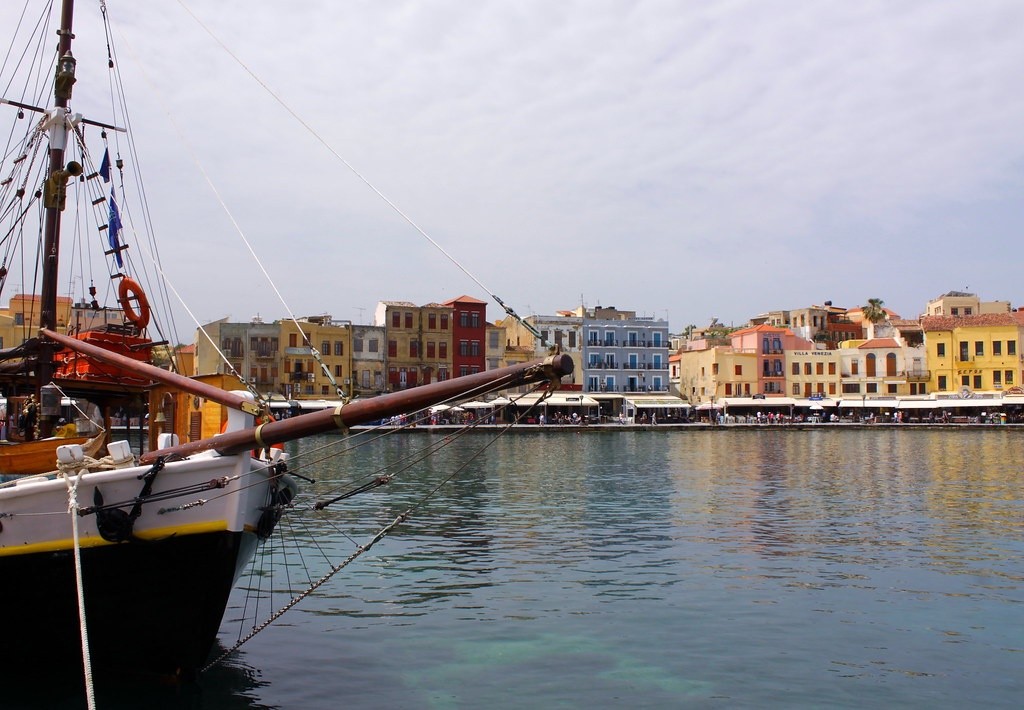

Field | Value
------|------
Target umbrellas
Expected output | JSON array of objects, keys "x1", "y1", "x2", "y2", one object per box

[{"x1": 810, "y1": 402, "x2": 824, "y2": 413}]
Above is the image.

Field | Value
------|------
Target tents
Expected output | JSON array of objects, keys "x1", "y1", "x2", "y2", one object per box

[{"x1": 460, "y1": 401, "x2": 494, "y2": 424}]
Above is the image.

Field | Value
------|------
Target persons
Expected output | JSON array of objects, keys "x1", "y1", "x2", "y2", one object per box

[
  {"x1": 1002, "y1": 410, "x2": 1005, "y2": 413},
  {"x1": 929, "y1": 409, "x2": 936, "y2": 423},
  {"x1": 990, "y1": 412, "x2": 995, "y2": 424},
  {"x1": 863, "y1": 412, "x2": 868, "y2": 419},
  {"x1": 641, "y1": 411, "x2": 646, "y2": 419},
  {"x1": 1007, "y1": 412, "x2": 1010, "y2": 423},
  {"x1": 831, "y1": 413, "x2": 837, "y2": 423},
  {"x1": 534, "y1": 413, "x2": 544, "y2": 426},
  {"x1": 619, "y1": 411, "x2": 625, "y2": 424},
  {"x1": 554, "y1": 412, "x2": 562, "y2": 424},
  {"x1": 490, "y1": 410, "x2": 497, "y2": 425},
  {"x1": 572, "y1": 412, "x2": 581, "y2": 424},
  {"x1": 762, "y1": 412, "x2": 765, "y2": 420},
  {"x1": 652, "y1": 413, "x2": 657, "y2": 425},
  {"x1": 1015, "y1": 412, "x2": 1021, "y2": 419},
  {"x1": 757, "y1": 411, "x2": 761, "y2": 423},
  {"x1": 55, "y1": 417, "x2": 80, "y2": 438},
  {"x1": 566, "y1": 415, "x2": 573, "y2": 424},
  {"x1": 431, "y1": 414, "x2": 440, "y2": 426},
  {"x1": 463, "y1": 411, "x2": 474, "y2": 425},
  {"x1": 942, "y1": 409, "x2": 950, "y2": 423},
  {"x1": 893, "y1": 410, "x2": 905, "y2": 423},
  {"x1": 484, "y1": 413, "x2": 488, "y2": 424},
  {"x1": 822, "y1": 409, "x2": 827, "y2": 423},
  {"x1": 717, "y1": 411, "x2": 720, "y2": 421},
  {"x1": 767, "y1": 411, "x2": 774, "y2": 423},
  {"x1": 390, "y1": 414, "x2": 407, "y2": 429},
  {"x1": 869, "y1": 411, "x2": 874, "y2": 419},
  {"x1": 775, "y1": 410, "x2": 784, "y2": 424},
  {"x1": 799, "y1": 411, "x2": 804, "y2": 420},
  {"x1": 114, "y1": 404, "x2": 128, "y2": 424},
  {"x1": 919, "y1": 415, "x2": 922, "y2": 422},
  {"x1": 745, "y1": 412, "x2": 753, "y2": 424}
]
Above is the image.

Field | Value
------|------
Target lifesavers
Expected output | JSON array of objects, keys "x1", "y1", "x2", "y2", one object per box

[{"x1": 118, "y1": 278, "x2": 150, "y2": 326}]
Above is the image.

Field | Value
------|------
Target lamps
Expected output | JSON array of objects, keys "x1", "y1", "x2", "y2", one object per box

[{"x1": 154, "y1": 404, "x2": 168, "y2": 423}]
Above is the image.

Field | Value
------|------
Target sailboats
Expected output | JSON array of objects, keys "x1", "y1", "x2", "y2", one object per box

[{"x1": 0, "y1": 2, "x2": 580, "y2": 666}]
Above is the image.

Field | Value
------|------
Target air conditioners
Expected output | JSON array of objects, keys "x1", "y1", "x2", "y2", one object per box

[
  {"x1": 639, "y1": 342, "x2": 643, "y2": 346},
  {"x1": 639, "y1": 373, "x2": 645, "y2": 378},
  {"x1": 248, "y1": 377, "x2": 255, "y2": 382},
  {"x1": 294, "y1": 388, "x2": 300, "y2": 392},
  {"x1": 344, "y1": 380, "x2": 350, "y2": 384},
  {"x1": 250, "y1": 384, "x2": 256, "y2": 389},
  {"x1": 308, "y1": 378, "x2": 315, "y2": 382},
  {"x1": 308, "y1": 373, "x2": 315, "y2": 377},
  {"x1": 294, "y1": 383, "x2": 300, "y2": 387}
]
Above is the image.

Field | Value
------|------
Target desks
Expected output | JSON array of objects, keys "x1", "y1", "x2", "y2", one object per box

[
  {"x1": 910, "y1": 416, "x2": 969, "y2": 422},
  {"x1": 727, "y1": 415, "x2": 757, "y2": 423}
]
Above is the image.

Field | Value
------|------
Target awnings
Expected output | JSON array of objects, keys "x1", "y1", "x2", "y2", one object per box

[
  {"x1": 511, "y1": 396, "x2": 547, "y2": 406},
  {"x1": 795, "y1": 398, "x2": 1024, "y2": 409},
  {"x1": 720, "y1": 397, "x2": 799, "y2": 418},
  {"x1": 488, "y1": 397, "x2": 514, "y2": 406},
  {"x1": 635, "y1": 403, "x2": 692, "y2": 408},
  {"x1": 545, "y1": 394, "x2": 599, "y2": 406},
  {"x1": 434, "y1": 404, "x2": 464, "y2": 412}
]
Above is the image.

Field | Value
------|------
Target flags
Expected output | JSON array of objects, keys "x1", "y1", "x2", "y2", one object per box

[
  {"x1": 99, "y1": 146, "x2": 111, "y2": 182},
  {"x1": 108, "y1": 186, "x2": 124, "y2": 269}
]
